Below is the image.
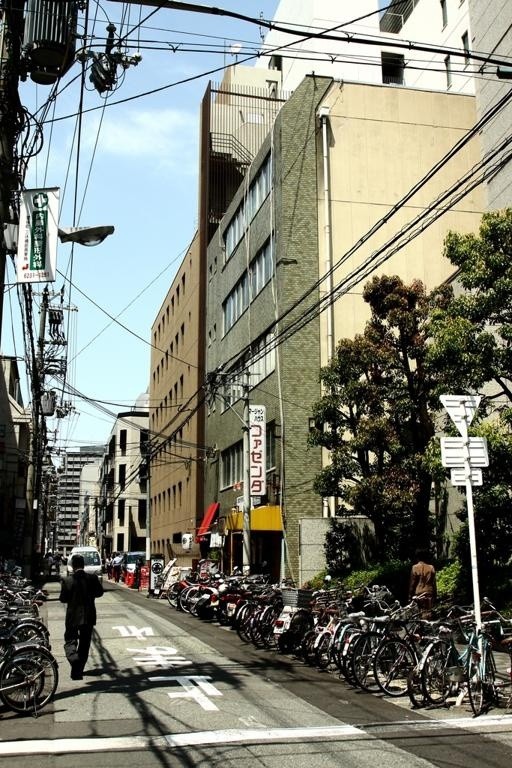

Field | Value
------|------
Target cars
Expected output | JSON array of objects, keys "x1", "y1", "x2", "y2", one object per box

[{"x1": 124, "y1": 549, "x2": 146, "y2": 575}]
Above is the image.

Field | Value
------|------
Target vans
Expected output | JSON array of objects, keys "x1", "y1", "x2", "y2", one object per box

[{"x1": 61, "y1": 546, "x2": 108, "y2": 587}]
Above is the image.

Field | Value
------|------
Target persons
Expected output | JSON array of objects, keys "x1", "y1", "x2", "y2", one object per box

[
  {"x1": 133, "y1": 557, "x2": 144, "y2": 589},
  {"x1": 101, "y1": 552, "x2": 128, "y2": 583},
  {"x1": 409, "y1": 549, "x2": 438, "y2": 642},
  {"x1": 43, "y1": 552, "x2": 60, "y2": 578},
  {"x1": 60, "y1": 555, "x2": 103, "y2": 681}
]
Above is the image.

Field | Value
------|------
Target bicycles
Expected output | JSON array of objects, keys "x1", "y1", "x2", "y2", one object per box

[{"x1": 0, "y1": 562, "x2": 58, "y2": 722}]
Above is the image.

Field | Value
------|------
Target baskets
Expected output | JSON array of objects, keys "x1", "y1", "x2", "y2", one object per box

[
  {"x1": 459, "y1": 611, "x2": 499, "y2": 640},
  {"x1": 282, "y1": 587, "x2": 313, "y2": 607}
]
